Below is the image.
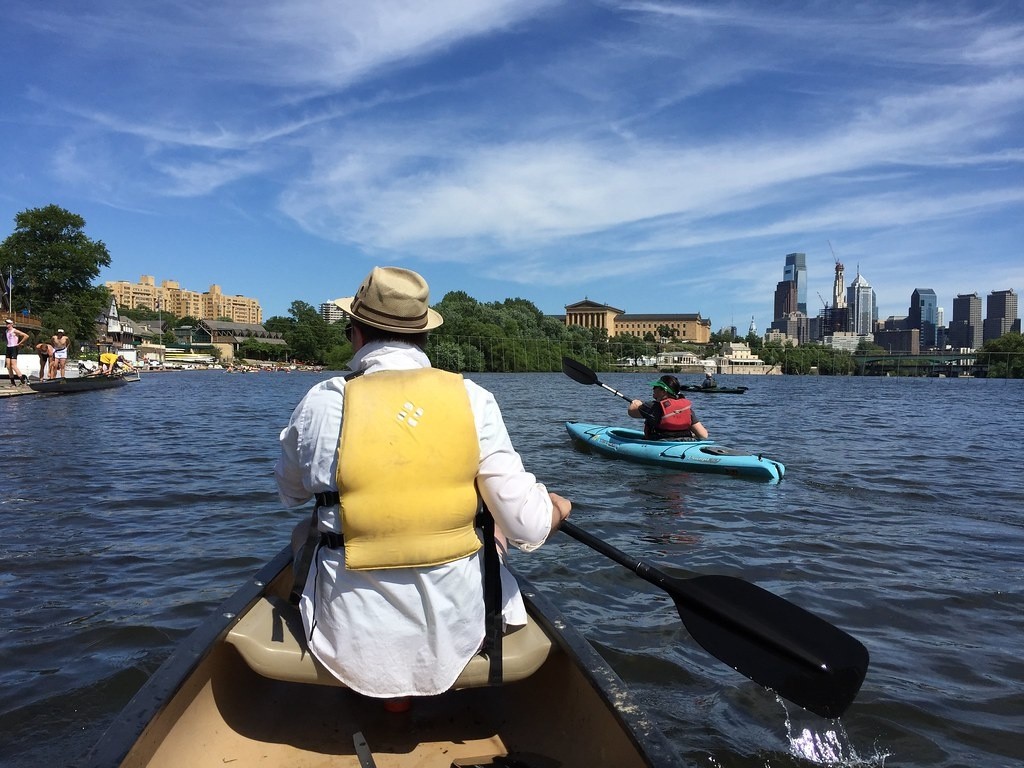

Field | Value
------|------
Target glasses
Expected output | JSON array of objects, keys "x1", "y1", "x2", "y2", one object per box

[{"x1": 345, "y1": 324, "x2": 358, "y2": 342}]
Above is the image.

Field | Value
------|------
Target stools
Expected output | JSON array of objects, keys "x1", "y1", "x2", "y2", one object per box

[{"x1": 225, "y1": 587, "x2": 552, "y2": 692}]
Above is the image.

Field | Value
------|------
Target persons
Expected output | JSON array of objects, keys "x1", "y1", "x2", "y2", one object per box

[
  {"x1": 96, "y1": 353, "x2": 133, "y2": 376},
  {"x1": 241, "y1": 367, "x2": 246, "y2": 372},
  {"x1": 272, "y1": 266, "x2": 572, "y2": 711},
  {"x1": 627, "y1": 374, "x2": 708, "y2": 440},
  {"x1": 227, "y1": 367, "x2": 232, "y2": 373},
  {"x1": 5, "y1": 317, "x2": 29, "y2": 386},
  {"x1": 148, "y1": 358, "x2": 150, "y2": 364},
  {"x1": 702, "y1": 373, "x2": 717, "y2": 389},
  {"x1": 36, "y1": 343, "x2": 54, "y2": 382},
  {"x1": 51, "y1": 329, "x2": 70, "y2": 379}
]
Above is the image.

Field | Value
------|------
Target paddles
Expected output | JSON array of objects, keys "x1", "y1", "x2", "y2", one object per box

[
  {"x1": 559, "y1": 520, "x2": 869, "y2": 720},
  {"x1": 563, "y1": 358, "x2": 633, "y2": 404}
]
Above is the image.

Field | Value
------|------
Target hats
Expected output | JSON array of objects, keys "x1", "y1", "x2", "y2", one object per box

[
  {"x1": 58, "y1": 329, "x2": 65, "y2": 333},
  {"x1": 706, "y1": 373, "x2": 712, "y2": 376},
  {"x1": 333, "y1": 266, "x2": 444, "y2": 333},
  {"x1": 5, "y1": 320, "x2": 13, "y2": 323},
  {"x1": 648, "y1": 378, "x2": 676, "y2": 396}
]
